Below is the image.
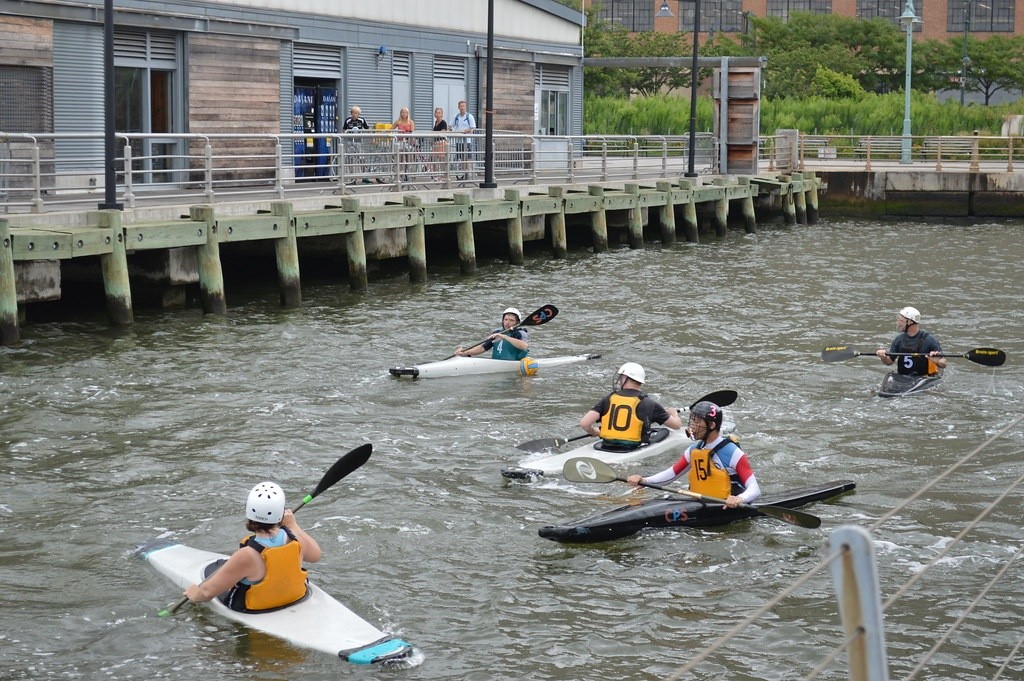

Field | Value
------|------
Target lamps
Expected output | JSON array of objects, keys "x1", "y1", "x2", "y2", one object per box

[{"x1": 375, "y1": 47, "x2": 387, "y2": 58}]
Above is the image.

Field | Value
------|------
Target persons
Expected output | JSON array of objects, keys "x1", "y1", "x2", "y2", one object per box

[
  {"x1": 343, "y1": 106, "x2": 373, "y2": 185},
  {"x1": 627, "y1": 401, "x2": 761, "y2": 510},
  {"x1": 579, "y1": 362, "x2": 682, "y2": 447},
  {"x1": 183, "y1": 482, "x2": 322, "y2": 611},
  {"x1": 448, "y1": 101, "x2": 476, "y2": 180},
  {"x1": 383, "y1": 108, "x2": 414, "y2": 182},
  {"x1": 430, "y1": 108, "x2": 448, "y2": 185},
  {"x1": 454, "y1": 307, "x2": 528, "y2": 360},
  {"x1": 876, "y1": 306, "x2": 947, "y2": 377}
]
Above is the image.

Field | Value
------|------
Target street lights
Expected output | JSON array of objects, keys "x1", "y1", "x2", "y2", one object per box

[
  {"x1": 655, "y1": 0, "x2": 700, "y2": 177},
  {"x1": 895, "y1": 0, "x2": 921, "y2": 164},
  {"x1": 957, "y1": 3, "x2": 971, "y2": 131}
]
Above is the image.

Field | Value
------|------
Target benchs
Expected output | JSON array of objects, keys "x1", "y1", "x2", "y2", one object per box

[
  {"x1": 580, "y1": 136, "x2": 692, "y2": 158},
  {"x1": 798, "y1": 135, "x2": 975, "y2": 161}
]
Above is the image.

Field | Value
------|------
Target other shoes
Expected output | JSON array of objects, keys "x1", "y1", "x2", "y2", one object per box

[{"x1": 362, "y1": 179, "x2": 373, "y2": 184}]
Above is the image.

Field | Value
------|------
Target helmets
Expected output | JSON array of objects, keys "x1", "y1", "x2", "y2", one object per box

[
  {"x1": 617, "y1": 361, "x2": 646, "y2": 385},
  {"x1": 246, "y1": 481, "x2": 285, "y2": 524},
  {"x1": 501, "y1": 307, "x2": 522, "y2": 326},
  {"x1": 899, "y1": 306, "x2": 920, "y2": 324},
  {"x1": 693, "y1": 400, "x2": 723, "y2": 429}
]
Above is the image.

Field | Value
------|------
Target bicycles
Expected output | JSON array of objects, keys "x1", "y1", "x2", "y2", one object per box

[
  {"x1": 331, "y1": 149, "x2": 396, "y2": 184},
  {"x1": 413, "y1": 144, "x2": 466, "y2": 179}
]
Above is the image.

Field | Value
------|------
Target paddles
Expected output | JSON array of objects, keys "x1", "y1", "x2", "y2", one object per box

[
  {"x1": 421, "y1": 304, "x2": 559, "y2": 365},
  {"x1": 515, "y1": 389, "x2": 739, "y2": 452},
  {"x1": 562, "y1": 456, "x2": 823, "y2": 529},
  {"x1": 166, "y1": 440, "x2": 375, "y2": 615},
  {"x1": 821, "y1": 343, "x2": 1008, "y2": 367}
]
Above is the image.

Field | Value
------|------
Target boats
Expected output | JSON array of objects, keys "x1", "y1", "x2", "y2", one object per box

[
  {"x1": 388, "y1": 350, "x2": 601, "y2": 379},
  {"x1": 538, "y1": 480, "x2": 857, "y2": 545},
  {"x1": 878, "y1": 368, "x2": 946, "y2": 398},
  {"x1": 500, "y1": 422, "x2": 737, "y2": 479},
  {"x1": 139, "y1": 542, "x2": 412, "y2": 666}
]
